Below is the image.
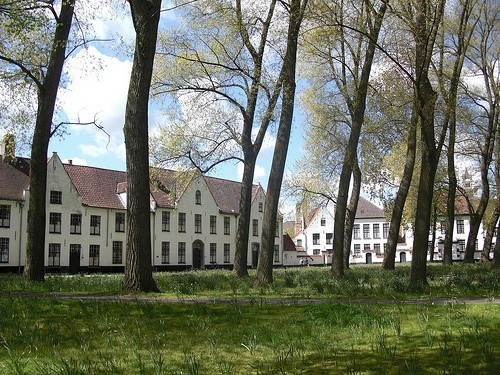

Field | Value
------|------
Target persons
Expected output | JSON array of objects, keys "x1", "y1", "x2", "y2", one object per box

[
  {"x1": 300, "y1": 257, "x2": 304, "y2": 267},
  {"x1": 304, "y1": 257, "x2": 308, "y2": 267}
]
